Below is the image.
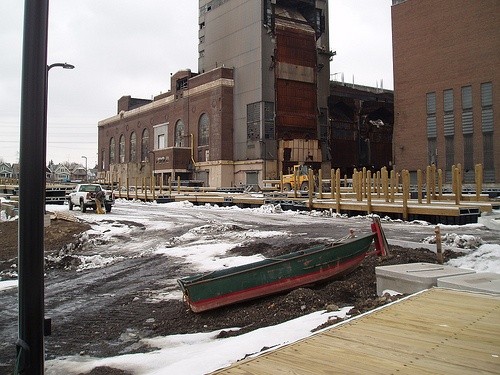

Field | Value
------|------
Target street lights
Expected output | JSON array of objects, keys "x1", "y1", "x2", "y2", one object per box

[{"x1": 82, "y1": 156, "x2": 88, "y2": 181}]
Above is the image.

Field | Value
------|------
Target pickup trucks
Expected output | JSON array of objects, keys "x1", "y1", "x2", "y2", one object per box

[{"x1": 67, "y1": 183, "x2": 115, "y2": 214}]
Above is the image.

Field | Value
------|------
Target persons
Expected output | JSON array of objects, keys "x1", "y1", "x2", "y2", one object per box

[{"x1": 95, "y1": 191, "x2": 105, "y2": 214}]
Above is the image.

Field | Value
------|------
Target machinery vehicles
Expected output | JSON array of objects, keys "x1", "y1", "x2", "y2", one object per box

[{"x1": 282, "y1": 164, "x2": 330, "y2": 193}]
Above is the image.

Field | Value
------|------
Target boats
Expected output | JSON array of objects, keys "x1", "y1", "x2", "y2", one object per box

[{"x1": 177, "y1": 229, "x2": 377, "y2": 312}]
